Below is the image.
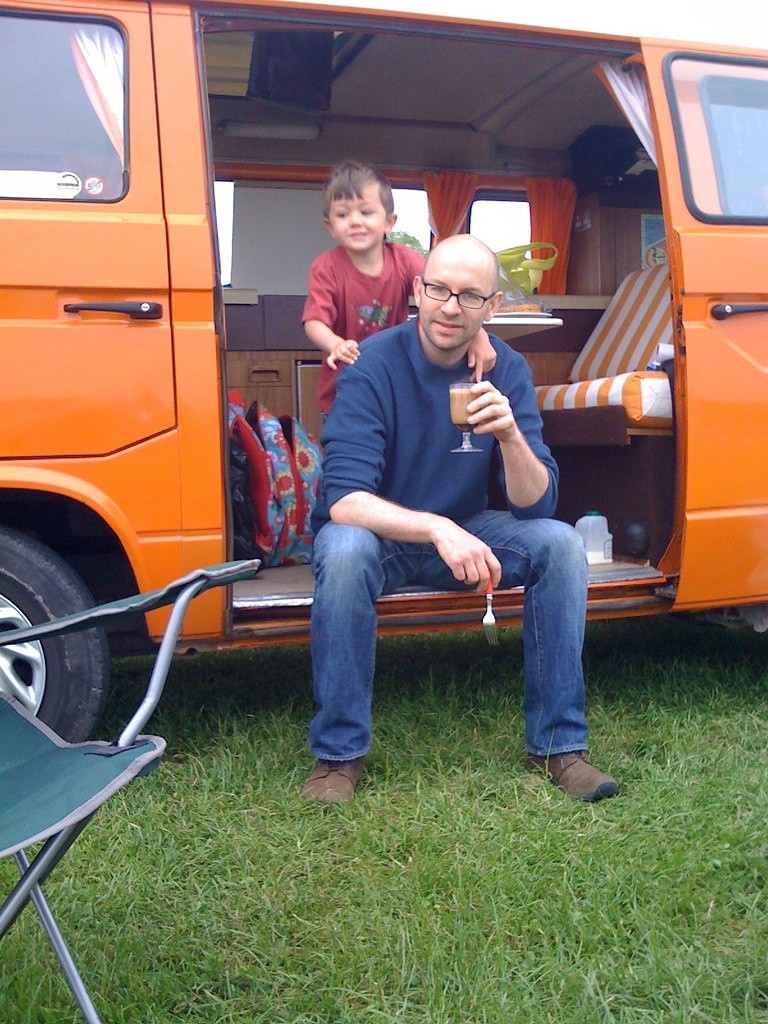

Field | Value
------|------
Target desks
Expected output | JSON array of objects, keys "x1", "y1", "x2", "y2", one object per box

[{"x1": 408, "y1": 311, "x2": 563, "y2": 341}]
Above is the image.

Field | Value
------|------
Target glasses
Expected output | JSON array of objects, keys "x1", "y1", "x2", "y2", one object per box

[{"x1": 421, "y1": 276, "x2": 497, "y2": 310}]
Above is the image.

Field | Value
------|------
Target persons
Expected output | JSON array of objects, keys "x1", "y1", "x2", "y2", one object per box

[
  {"x1": 303, "y1": 157, "x2": 495, "y2": 504},
  {"x1": 303, "y1": 234, "x2": 618, "y2": 817}
]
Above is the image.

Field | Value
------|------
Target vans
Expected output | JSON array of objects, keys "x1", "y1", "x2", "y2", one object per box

[{"x1": 2, "y1": 1, "x2": 767, "y2": 751}]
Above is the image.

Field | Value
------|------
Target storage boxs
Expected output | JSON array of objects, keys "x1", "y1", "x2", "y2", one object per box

[{"x1": 225, "y1": 291, "x2": 323, "y2": 352}]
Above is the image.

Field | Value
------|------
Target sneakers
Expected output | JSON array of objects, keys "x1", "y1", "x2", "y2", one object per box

[
  {"x1": 300, "y1": 754, "x2": 364, "y2": 807},
  {"x1": 526, "y1": 750, "x2": 619, "y2": 802}
]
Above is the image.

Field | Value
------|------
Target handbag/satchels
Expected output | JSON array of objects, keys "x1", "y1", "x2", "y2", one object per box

[{"x1": 494, "y1": 242, "x2": 558, "y2": 313}]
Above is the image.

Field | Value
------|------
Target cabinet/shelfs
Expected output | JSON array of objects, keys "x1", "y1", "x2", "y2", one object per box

[{"x1": 225, "y1": 350, "x2": 298, "y2": 425}]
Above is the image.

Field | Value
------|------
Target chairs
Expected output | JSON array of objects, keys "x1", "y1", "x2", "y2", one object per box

[
  {"x1": 0, "y1": 557, "x2": 258, "y2": 1023},
  {"x1": 528, "y1": 264, "x2": 678, "y2": 451}
]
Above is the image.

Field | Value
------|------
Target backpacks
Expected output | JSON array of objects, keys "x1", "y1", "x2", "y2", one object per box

[{"x1": 227, "y1": 398, "x2": 323, "y2": 570}]
